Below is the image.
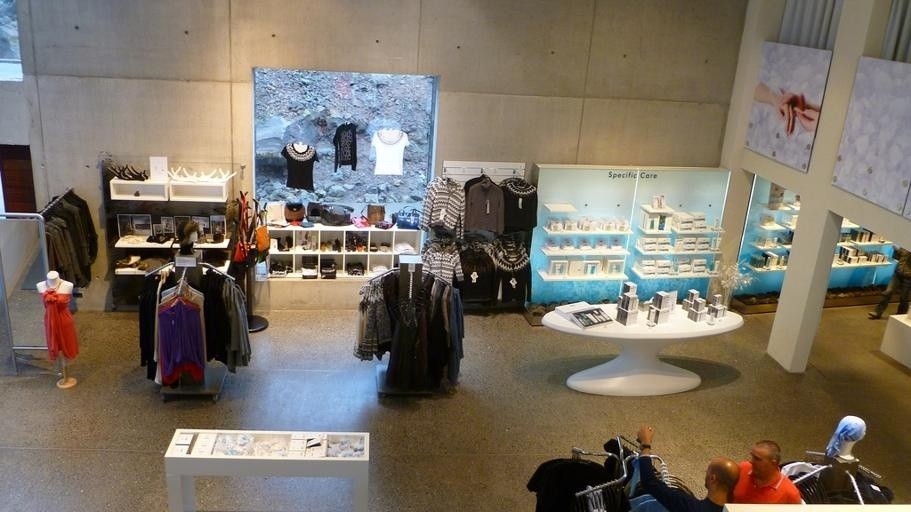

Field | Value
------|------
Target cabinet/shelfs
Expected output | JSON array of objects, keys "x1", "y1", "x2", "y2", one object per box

[
  {"x1": 101, "y1": 157, "x2": 239, "y2": 311},
  {"x1": 718, "y1": 174, "x2": 899, "y2": 296},
  {"x1": 530, "y1": 163, "x2": 734, "y2": 303},
  {"x1": 264, "y1": 219, "x2": 424, "y2": 282},
  {"x1": 164, "y1": 427, "x2": 368, "y2": 510}
]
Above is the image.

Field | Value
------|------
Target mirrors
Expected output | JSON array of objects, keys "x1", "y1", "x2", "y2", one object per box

[{"x1": 0, "y1": 218, "x2": 49, "y2": 348}]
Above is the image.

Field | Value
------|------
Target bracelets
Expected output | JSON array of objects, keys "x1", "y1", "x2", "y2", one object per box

[{"x1": 640, "y1": 444, "x2": 651, "y2": 450}]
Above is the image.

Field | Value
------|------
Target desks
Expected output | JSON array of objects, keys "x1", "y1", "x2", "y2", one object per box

[{"x1": 542, "y1": 299, "x2": 745, "y2": 396}]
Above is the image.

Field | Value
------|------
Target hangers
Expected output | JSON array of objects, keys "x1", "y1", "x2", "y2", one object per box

[{"x1": 585, "y1": 462, "x2": 693, "y2": 511}]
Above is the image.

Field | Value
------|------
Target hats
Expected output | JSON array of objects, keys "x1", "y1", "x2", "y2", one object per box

[{"x1": 177, "y1": 220, "x2": 200, "y2": 245}]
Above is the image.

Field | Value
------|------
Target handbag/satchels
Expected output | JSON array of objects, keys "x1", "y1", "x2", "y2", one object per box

[
  {"x1": 367, "y1": 204, "x2": 419, "y2": 228},
  {"x1": 228, "y1": 195, "x2": 270, "y2": 280},
  {"x1": 302, "y1": 257, "x2": 337, "y2": 279},
  {"x1": 285, "y1": 203, "x2": 354, "y2": 225}
]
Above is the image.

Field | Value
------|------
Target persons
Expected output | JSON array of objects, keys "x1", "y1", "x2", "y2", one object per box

[
  {"x1": 756, "y1": 79, "x2": 820, "y2": 138},
  {"x1": 36, "y1": 270, "x2": 78, "y2": 362},
  {"x1": 869, "y1": 248, "x2": 911, "y2": 318},
  {"x1": 734, "y1": 439, "x2": 801, "y2": 503},
  {"x1": 638, "y1": 427, "x2": 742, "y2": 511}
]
